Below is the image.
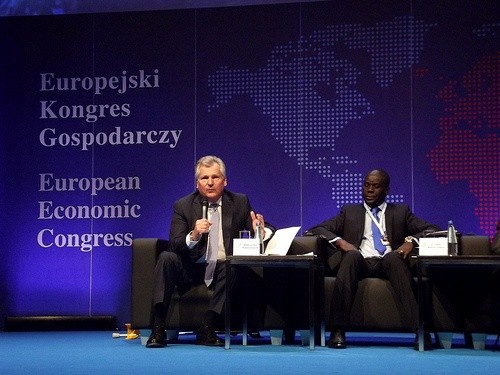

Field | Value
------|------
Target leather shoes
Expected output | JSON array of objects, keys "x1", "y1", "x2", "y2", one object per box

[
  {"x1": 196, "y1": 325, "x2": 226, "y2": 346},
  {"x1": 327, "y1": 328, "x2": 347, "y2": 349},
  {"x1": 146, "y1": 324, "x2": 167, "y2": 347},
  {"x1": 414, "y1": 329, "x2": 432, "y2": 351}
]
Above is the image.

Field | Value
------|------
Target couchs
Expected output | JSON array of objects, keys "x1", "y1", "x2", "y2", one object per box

[
  {"x1": 131, "y1": 238, "x2": 295, "y2": 347},
  {"x1": 291, "y1": 234, "x2": 489, "y2": 351}
]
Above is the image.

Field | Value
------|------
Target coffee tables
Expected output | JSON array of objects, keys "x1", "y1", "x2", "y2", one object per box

[
  {"x1": 224, "y1": 255, "x2": 327, "y2": 351},
  {"x1": 417, "y1": 254, "x2": 500, "y2": 351}
]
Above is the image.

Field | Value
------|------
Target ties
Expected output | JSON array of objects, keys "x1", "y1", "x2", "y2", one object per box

[
  {"x1": 370, "y1": 207, "x2": 387, "y2": 255},
  {"x1": 204, "y1": 197, "x2": 223, "y2": 288}
]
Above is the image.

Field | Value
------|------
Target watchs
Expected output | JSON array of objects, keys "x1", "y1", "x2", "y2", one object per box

[
  {"x1": 404, "y1": 236, "x2": 416, "y2": 247},
  {"x1": 188, "y1": 230, "x2": 201, "y2": 241}
]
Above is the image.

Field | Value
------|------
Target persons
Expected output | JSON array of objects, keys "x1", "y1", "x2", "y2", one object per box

[
  {"x1": 145, "y1": 155, "x2": 275, "y2": 347},
  {"x1": 307, "y1": 168, "x2": 435, "y2": 352}
]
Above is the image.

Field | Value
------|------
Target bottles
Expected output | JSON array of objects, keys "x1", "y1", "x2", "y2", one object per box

[
  {"x1": 448, "y1": 220, "x2": 458, "y2": 256},
  {"x1": 254, "y1": 221, "x2": 264, "y2": 254}
]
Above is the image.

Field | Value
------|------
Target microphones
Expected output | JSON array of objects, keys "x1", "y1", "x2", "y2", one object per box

[{"x1": 202, "y1": 199, "x2": 208, "y2": 219}]
